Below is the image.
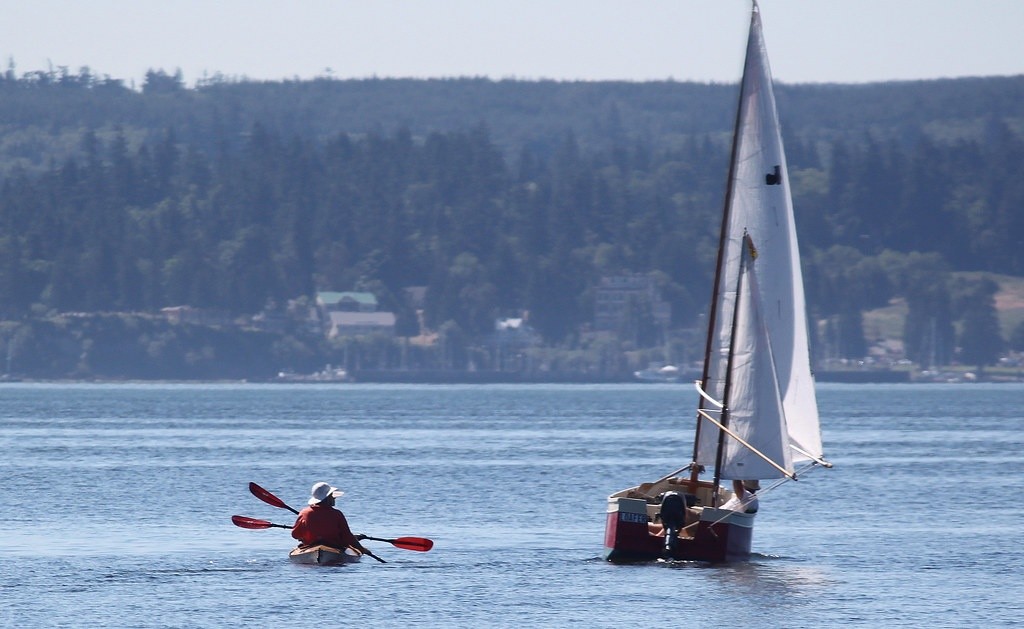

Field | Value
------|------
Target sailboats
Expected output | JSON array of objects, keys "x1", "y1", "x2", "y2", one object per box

[{"x1": 605, "y1": 3, "x2": 832, "y2": 568}]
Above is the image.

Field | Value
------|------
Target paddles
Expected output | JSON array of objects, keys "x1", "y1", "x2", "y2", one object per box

[
  {"x1": 231, "y1": 514, "x2": 434, "y2": 552},
  {"x1": 637, "y1": 464, "x2": 690, "y2": 495},
  {"x1": 247, "y1": 480, "x2": 387, "y2": 564}
]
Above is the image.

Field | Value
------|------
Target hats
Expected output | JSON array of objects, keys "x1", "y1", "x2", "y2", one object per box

[
  {"x1": 308, "y1": 482, "x2": 338, "y2": 505},
  {"x1": 741, "y1": 480, "x2": 761, "y2": 489}
]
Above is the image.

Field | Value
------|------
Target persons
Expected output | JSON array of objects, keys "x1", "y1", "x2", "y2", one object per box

[
  {"x1": 291, "y1": 482, "x2": 371, "y2": 554},
  {"x1": 647, "y1": 480, "x2": 760, "y2": 536}
]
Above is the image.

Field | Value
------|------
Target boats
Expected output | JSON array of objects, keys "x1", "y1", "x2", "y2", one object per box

[{"x1": 288, "y1": 544, "x2": 363, "y2": 566}]
